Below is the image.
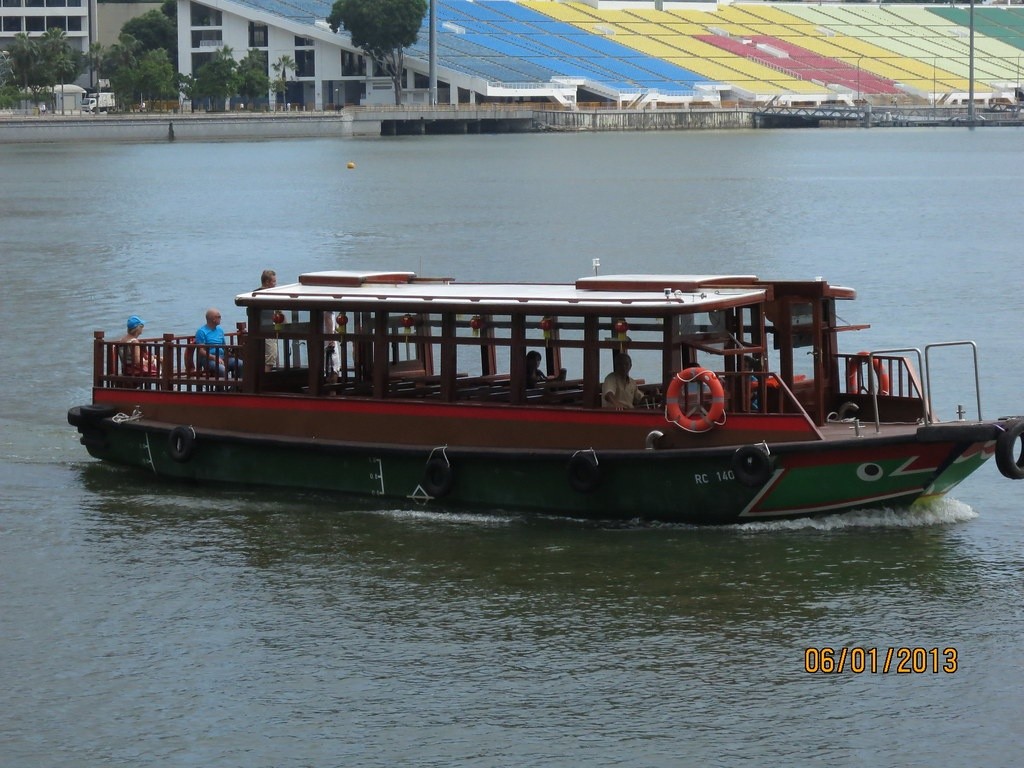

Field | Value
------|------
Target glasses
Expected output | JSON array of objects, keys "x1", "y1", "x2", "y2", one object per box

[
  {"x1": 140, "y1": 324, "x2": 144, "y2": 328},
  {"x1": 215, "y1": 315, "x2": 222, "y2": 319}
]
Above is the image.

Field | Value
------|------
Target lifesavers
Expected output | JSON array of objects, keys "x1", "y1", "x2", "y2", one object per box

[
  {"x1": 731, "y1": 445, "x2": 776, "y2": 487},
  {"x1": 848, "y1": 352, "x2": 889, "y2": 396},
  {"x1": 169, "y1": 426, "x2": 195, "y2": 459},
  {"x1": 80, "y1": 404, "x2": 118, "y2": 419},
  {"x1": 666, "y1": 367, "x2": 726, "y2": 433},
  {"x1": 566, "y1": 454, "x2": 605, "y2": 498},
  {"x1": 995, "y1": 418, "x2": 1024, "y2": 480},
  {"x1": 419, "y1": 453, "x2": 460, "y2": 499}
]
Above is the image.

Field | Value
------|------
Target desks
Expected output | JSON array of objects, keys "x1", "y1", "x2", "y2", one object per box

[
  {"x1": 406, "y1": 370, "x2": 468, "y2": 388},
  {"x1": 482, "y1": 378, "x2": 510, "y2": 387}
]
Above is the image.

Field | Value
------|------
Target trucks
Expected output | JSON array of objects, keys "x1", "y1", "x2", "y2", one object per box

[{"x1": 80, "y1": 93, "x2": 115, "y2": 112}]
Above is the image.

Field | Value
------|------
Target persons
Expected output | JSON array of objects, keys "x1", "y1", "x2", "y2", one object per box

[
  {"x1": 601, "y1": 354, "x2": 648, "y2": 411},
  {"x1": 119, "y1": 316, "x2": 176, "y2": 391},
  {"x1": 724, "y1": 357, "x2": 759, "y2": 410},
  {"x1": 41, "y1": 103, "x2": 46, "y2": 115},
  {"x1": 240, "y1": 102, "x2": 244, "y2": 110},
  {"x1": 525, "y1": 350, "x2": 564, "y2": 383},
  {"x1": 324, "y1": 311, "x2": 341, "y2": 377},
  {"x1": 683, "y1": 362, "x2": 708, "y2": 393},
  {"x1": 253, "y1": 270, "x2": 286, "y2": 371},
  {"x1": 139, "y1": 101, "x2": 145, "y2": 111},
  {"x1": 195, "y1": 309, "x2": 243, "y2": 392},
  {"x1": 286, "y1": 102, "x2": 290, "y2": 111}
]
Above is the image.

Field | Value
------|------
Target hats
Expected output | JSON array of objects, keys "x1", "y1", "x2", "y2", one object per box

[{"x1": 127, "y1": 316, "x2": 145, "y2": 330}]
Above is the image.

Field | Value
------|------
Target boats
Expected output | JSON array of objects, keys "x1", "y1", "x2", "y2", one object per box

[{"x1": 68, "y1": 270, "x2": 1024, "y2": 524}]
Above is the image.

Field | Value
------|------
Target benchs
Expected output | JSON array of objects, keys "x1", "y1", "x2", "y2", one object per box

[
  {"x1": 160, "y1": 368, "x2": 210, "y2": 377},
  {"x1": 260, "y1": 357, "x2": 663, "y2": 411}
]
